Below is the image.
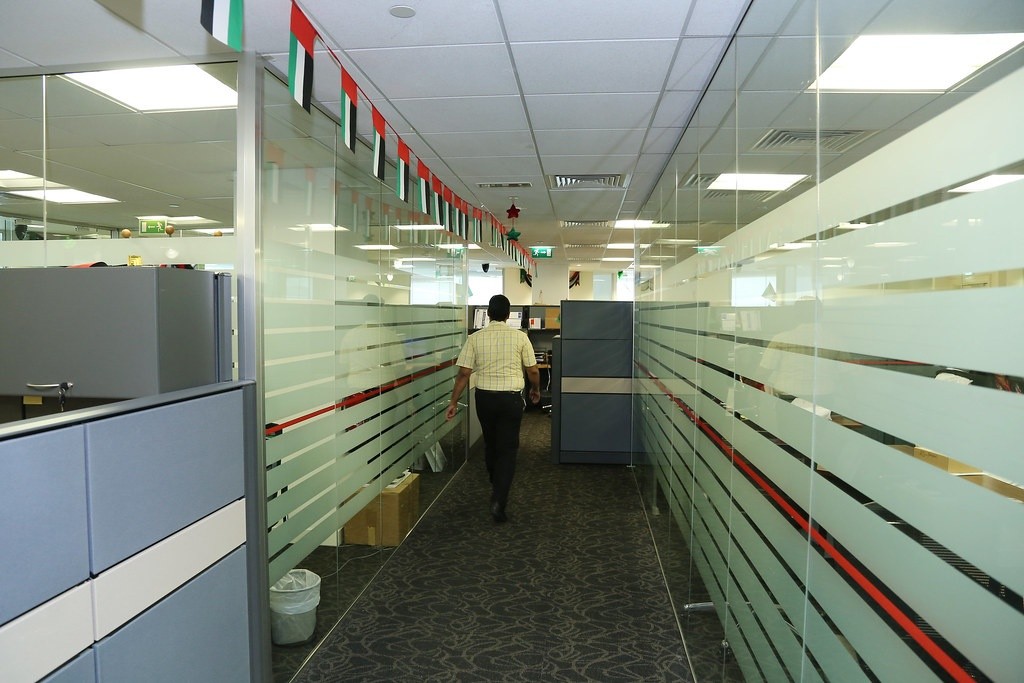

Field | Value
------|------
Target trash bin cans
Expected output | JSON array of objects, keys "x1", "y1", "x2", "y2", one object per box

[{"x1": 269, "y1": 568, "x2": 321, "y2": 648}]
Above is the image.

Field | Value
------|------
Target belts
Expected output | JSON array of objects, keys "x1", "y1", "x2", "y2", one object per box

[{"x1": 476, "y1": 388, "x2": 520, "y2": 395}]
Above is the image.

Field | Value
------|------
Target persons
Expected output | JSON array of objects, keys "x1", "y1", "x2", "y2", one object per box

[
  {"x1": 341, "y1": 295, "x2": 406, "y2": 395},
  {"x1": 761, "y1": 297, "x2": 850, "y2": 404},
  {"x1": 445, "y1": 295, "x2": 540, "y2": 520}
]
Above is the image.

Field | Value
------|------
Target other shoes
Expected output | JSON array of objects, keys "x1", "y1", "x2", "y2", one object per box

[
  {"x1": 490, "y1": 501, "x2": 507, "y2": 522},
  {"x1": 488, "y1": 482, "x2": 494, "y2": 496}
]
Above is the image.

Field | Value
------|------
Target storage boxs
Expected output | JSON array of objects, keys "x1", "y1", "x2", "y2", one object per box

[{"x1": 343, "y1": 474, "x2": 420, "y2": 545}]
